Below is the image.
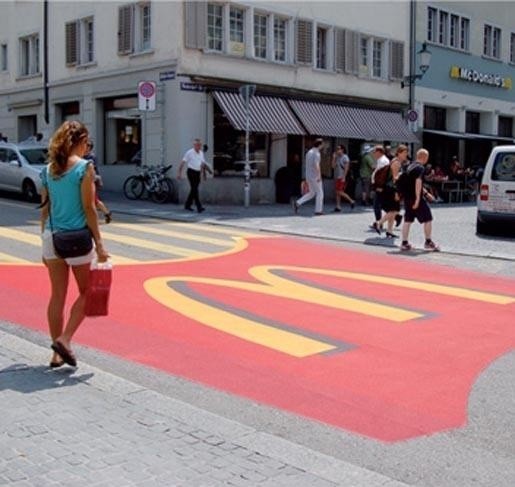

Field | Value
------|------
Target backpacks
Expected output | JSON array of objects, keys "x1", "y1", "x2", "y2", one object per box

[
  {"x1": 373, "y1": 158, "x2": 400, "y2": 188},
  {"x1": 395, "y1": 163, "x2": 421, "y2": 201}
]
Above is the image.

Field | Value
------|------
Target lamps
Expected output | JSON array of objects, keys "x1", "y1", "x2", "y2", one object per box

[{"x1": 400, "y1": 40, "x2": 432, "y2": 89}]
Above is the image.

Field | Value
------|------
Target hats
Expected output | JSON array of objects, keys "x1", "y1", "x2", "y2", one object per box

[{"x1": 360, "y1": 145, "x2": 374, "y2": 153}]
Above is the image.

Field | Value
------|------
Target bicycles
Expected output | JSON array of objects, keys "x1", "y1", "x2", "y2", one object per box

[{"x1": 121, "y1": 164, "x2": 175, "y2": 203}]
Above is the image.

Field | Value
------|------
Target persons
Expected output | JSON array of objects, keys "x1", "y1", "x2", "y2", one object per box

[
  {"x1": 177, "y1": 139, "x2": 206, "y2": 213},
  {"x1": 42, "y1": 120, "x2": 110, "y2": 367},
  {"x1": 355, "y1": 144, "x2": 484, "y2": 251},
  {"x1": 83, "y1": 141, "x2": 111, "y2": 224},
  {"x1": 331, "y1": 144, "x2": 356, "y2": 212},
  {"x1": 293, "y1": 138, "x2": 324, "y2": 215}
]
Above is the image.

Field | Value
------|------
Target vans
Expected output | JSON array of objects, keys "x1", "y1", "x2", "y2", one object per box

[{"x1": 475, "y1": 145, "x2": 515, "y2": 226}]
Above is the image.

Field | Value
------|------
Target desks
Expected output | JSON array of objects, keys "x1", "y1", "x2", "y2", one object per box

[{"x1": 433, "y1": 179, "x2": 471, "y2": 205}]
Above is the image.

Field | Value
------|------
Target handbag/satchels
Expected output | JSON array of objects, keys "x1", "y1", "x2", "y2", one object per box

[
  {"x1": 82, "y1": 255, "x2": 114, "y2": 318},
  {"x1": 344, "y1": 171, "x2": 356, "y2": 194},
  {"x1": 47, "y1": 225, "x2": 95, "y2": 260}
]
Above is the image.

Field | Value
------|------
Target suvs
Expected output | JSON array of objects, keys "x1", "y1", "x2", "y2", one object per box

[{"x1": 0, "y1": 139, "x2": 50, "y2": 203}]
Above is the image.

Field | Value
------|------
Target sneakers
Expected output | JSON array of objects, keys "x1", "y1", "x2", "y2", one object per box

[
  {"x1": 395, "y1": 214, "x2": 403, "y2": 227},
  {"x1": 423, "y1": 240, "x2": 441, "y2": 249},
  {"x1": 184, "y1": 206, "x2": 194, "y2": 211},
  {"x1": 334, "y1": 208, "x2": 341, "y2": 211},
  {"x1": 400, "y1": 244, "x2": 412, "y2": 251},
  {"x1": 386, "y1": 231, "x2": 399, "y2": 238},
  {"x1": 49, "y1": 338, "x2": 79, "y2": 368},
  {"x1": 314, "y1": 212, "x2": 325, "y2": 215},
  {"x1": 362, "y1": 200, "x2": 371, "y2": 206},
  {"x1": 369, "y1": 221, "x2": 386, "y2": 235},
  {"x1": 197, "y1": 207, "x2": 205, "y2": 212},
  {"x1": 103, "y1": 211, "x2": 112, "y2": 225},
  {"x1": 292, "y1": 199, "x2": 299, "y2": 214},
  {"x1": 351, "y1": 200, "x2": 356, "y2": 208}
]
